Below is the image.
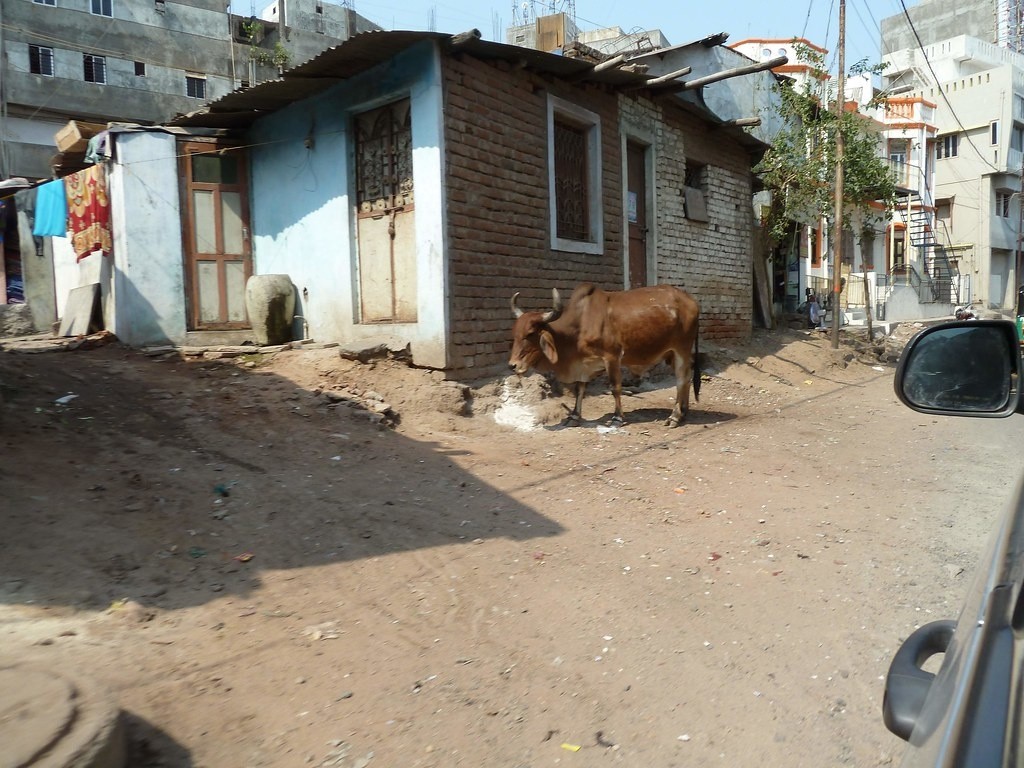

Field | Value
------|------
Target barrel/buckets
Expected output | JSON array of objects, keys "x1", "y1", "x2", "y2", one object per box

[{"x1": 838, "y1": 309, "x2": 844, "y2": 327}]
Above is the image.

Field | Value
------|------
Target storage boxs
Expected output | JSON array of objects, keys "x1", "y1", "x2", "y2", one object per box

[{"x1": 54, "y1": 120, "x2": 109, "y2": 153}]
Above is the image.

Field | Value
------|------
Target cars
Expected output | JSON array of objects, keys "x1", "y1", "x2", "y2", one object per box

[{"x1": 879, "y1": 315, "x2": 1023, "y2": 767}]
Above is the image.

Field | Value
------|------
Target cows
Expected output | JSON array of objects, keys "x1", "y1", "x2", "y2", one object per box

[{"x1": 507, "y1": 282, "x2": 703, "y2": 428}]
